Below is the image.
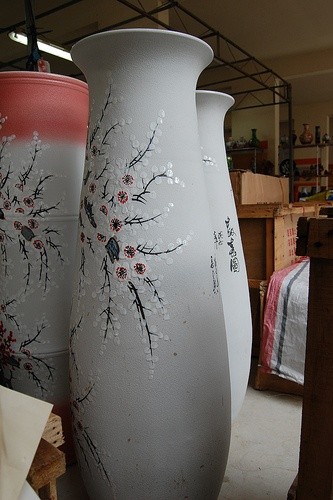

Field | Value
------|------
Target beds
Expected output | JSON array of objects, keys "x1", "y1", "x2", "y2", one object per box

[{"x1": 255, "y1": 255, "x2": 332, "y2": 395}]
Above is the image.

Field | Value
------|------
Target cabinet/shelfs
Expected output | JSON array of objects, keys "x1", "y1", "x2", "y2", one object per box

[
  {"x1": 280, "y1": 143, "x2": 332, "y2": 204},
  {"x1": 226, "y1": 146, "x2": 258, "y2": 173}
]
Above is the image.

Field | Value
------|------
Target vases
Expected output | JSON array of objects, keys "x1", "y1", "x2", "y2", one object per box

[
  {"x1": 195, "y1": 89, "x2": 253, "y2": 425},
  {"x1": 1, "y1": 70, "x2": 90, "y2": 467},
  {"x1": 236, "y1": 136, "x2": 247, "y2": 148},
  {"x1": 248, "y1": 128, "x2": 262, "y2": 148},
  {"x1": 291, "y1": 129, "x2": 297, "y2": 145},
  {"x1": 281, "y1": 135, "x2": 288, "y2": 145},
  {"x1": 299, "y1": 186, "x2": 310, "y2": 197},
  {"x1": 322, "y1": 132, "x2": 330, "y2": 144},
  {"x1": 311, "y1": 186, "x2": 321, "y2": 195},
  {"x1": 294, "y1": 184, "x2": 299, "y2": 202},
  {"x1": 69, "y1": 27, "x2": 231, "y2": 500},
  {"x1": 226, "y1": 137, "x2": 236, "y2": 148},
  {"x1": 315, "y1": 125, "x2": 321, "y2": 144},
  {"x1": 227, "y1": 157, "x2": 234, "y2": 171},
  {"x1": 299, "y1": 124, "x2": 313, "y2": 144}
]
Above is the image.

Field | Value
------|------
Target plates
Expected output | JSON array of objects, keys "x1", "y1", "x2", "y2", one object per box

[{"x1": 280, "y1": 158, "x2": 296, "y2": 175}]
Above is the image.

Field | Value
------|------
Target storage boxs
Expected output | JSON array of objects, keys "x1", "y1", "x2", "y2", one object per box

[{"x1": 230, "y1": 171, "x2": 290, "y2": 206}]
